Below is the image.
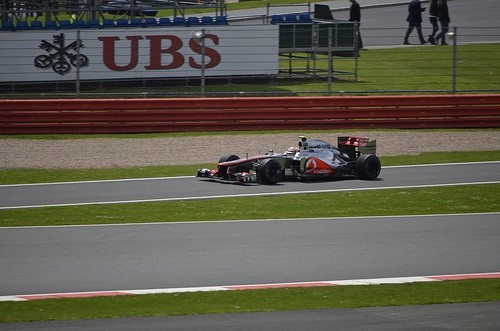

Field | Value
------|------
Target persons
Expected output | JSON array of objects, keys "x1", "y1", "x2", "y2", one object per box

[
  {"x1": 404, "y1": 0, "x2": 428, "y2": 45},
  {"x1": 430, "y1": 0, "x2": 450, "y2": 45},
  {"x1": 428, "y1": 0, "x2": 439, "y2": 41},
  {"x1": 349, "y1": 0, "x2": 363, "y2": 48}
]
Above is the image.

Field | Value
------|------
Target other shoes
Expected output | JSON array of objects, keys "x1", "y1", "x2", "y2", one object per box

[
  {"x1": 431, "y1": 38, "x2": 437, "y2": 45},
  {"x1": 421, "y1": 41, "x2": 428, "y2": 44},
  {"x1": 404, "y1": 41, "x2": 410, "y2": 44},
  {"x1": 428, "y1": 37, "x2": 432, "y2": 43},
  {"x1": 441, "y1": 41, "x2": 448, "y2": 45}
]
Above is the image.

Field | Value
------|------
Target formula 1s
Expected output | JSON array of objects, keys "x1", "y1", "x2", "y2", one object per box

[{"x1": 195, "y1": 135, "x2": 381, "y2": 185}]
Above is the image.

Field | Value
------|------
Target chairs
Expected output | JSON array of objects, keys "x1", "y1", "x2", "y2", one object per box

[
  {"x1": 286, "y1": 13, "x2": 297, "y2": 21},
  {"x1": 2, "y1": 16, "x2": 227, "y2": 30},
  {"x1": 272, "y1": 15, "x2": 283, "y2": 21},
  {"x1": 298, "y1": 140, "x2": 303, "y2": 150},
  {"x1": 299, "y1": 14, "x2": 310, "y2": 21}
]
